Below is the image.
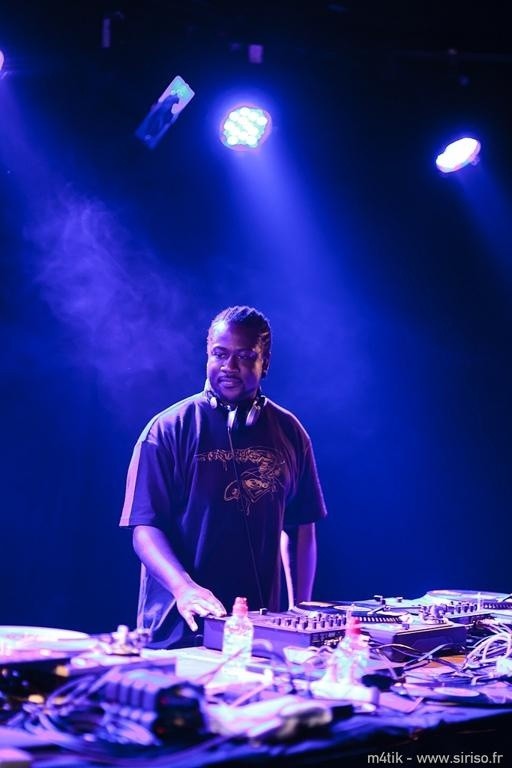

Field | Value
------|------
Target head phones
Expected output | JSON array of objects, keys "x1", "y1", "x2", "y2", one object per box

[{"x1": 203, "y1": 377, "x2": 266, "y2": 429}]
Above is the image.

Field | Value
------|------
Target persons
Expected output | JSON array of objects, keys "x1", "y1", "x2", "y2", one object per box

[{"x1": 119, "y1": 300, "x2": 328, "y2": 647}]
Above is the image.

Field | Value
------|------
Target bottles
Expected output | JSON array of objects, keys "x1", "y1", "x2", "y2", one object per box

[
  {"x1": 328, "y1": 613, "x2": 375, "y2": 686},
  {"x1": 221, "y1": 596, "x2": 254, "y2": 665}
]
